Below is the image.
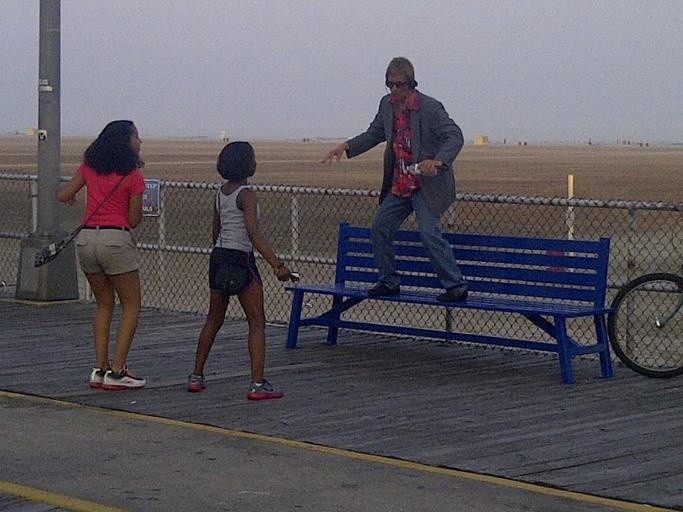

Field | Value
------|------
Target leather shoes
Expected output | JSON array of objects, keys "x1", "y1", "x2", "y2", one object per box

[
  {"x1": 436, "y1": 290, "x2": 468, "y2": 302},
  {"x1": 368, "y1": 283, "x2": 400, "y2": 295}
]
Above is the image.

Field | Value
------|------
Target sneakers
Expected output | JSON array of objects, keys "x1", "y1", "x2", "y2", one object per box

[
  {"x1": 247, "y1": 381, "x2": 283, "y2": 400},
  {"x1": 188, "y1": 375, "x2": 206, "y2": 391},
  {"x1": 89, "y1": 368, "x2": 110, "y2": 387},
  {"x1": 103, "y1": 368, "x2": 146, "y2": 389}
]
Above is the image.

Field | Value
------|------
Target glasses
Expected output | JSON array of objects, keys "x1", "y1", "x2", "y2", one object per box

[{"x1": 387, "y1": 81, "x2": 410, "y2": 88}]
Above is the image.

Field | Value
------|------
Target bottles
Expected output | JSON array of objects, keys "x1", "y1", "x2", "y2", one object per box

[{"x1": 403, "y1": 162, "x2": 440, "y2": 176}]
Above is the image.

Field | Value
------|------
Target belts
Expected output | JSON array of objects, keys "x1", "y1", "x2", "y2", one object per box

[{"x1": 81, "y1": 227, "x2": 131, "y2": 231}]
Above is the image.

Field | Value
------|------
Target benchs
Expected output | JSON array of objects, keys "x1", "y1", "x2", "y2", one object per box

[{"x1": 284, "y1": 220, "x2": 613, "y2": 386}]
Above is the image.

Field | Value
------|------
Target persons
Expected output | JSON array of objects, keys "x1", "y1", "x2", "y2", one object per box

[
  {"x1": 321, "y1": 57, "x2": 470, "y2": 302},
  {"x1": 54, "y1": 120, "x2": 147, "y2": 391},
  {"x1": 188, "y1": 142, "x2": 292, "y2": 400}
]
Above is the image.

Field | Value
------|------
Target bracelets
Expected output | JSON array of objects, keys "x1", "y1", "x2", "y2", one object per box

[{"x1": 274, "y1": 264, "x2": 284, "y2": 271}]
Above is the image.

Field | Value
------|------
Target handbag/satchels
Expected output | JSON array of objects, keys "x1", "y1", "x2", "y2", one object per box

[
  {"x1": 35, "y1": 240, "x2": 64, "y2": 266},
  {"x1": 214, "y1": 262, "x2": 248, "y2": 293}
]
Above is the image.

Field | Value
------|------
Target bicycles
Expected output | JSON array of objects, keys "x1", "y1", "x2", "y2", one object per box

[{"x1": 606, "y1": 272, "x2": 683, "y2": 378}]
